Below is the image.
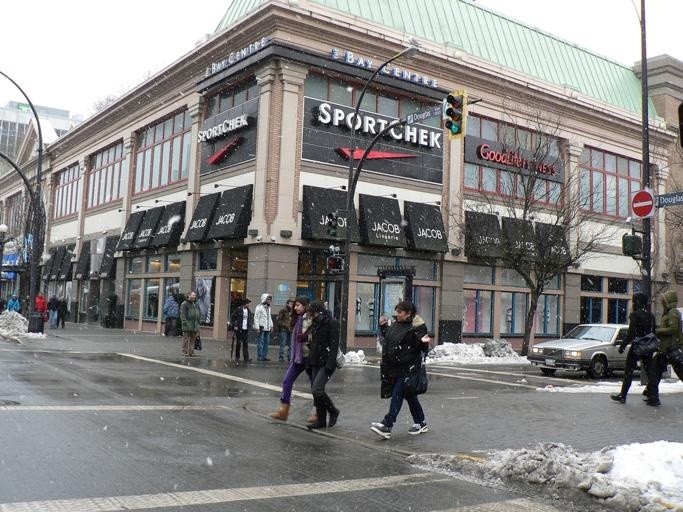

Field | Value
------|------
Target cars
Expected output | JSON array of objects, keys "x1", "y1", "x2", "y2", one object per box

[{"x1": 528, "y1": 323, "x2": 649, "y2": 381}]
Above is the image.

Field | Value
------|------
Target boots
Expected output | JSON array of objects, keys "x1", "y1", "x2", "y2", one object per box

[
  {"x1": 306, "y1": 406, "x2": 319, "y2": 423},
  {"x1": 328, "y1": 402, "x2": 338, "y2": 427},
  {"x1": 307, "y1": 411, "x2": 326, "y2": 429},
  {"x1": 270, "y1": 403, "x2": 290, "y2": 420}
]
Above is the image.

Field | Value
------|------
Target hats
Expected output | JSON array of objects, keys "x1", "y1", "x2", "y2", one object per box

[{"x1": 242, "y1": 299, "x2": 250, "y2": 306}]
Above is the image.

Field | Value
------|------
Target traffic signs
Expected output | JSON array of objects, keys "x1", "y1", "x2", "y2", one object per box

[{"x1": 654, "y1": 193, "x2": 683, "y2": 208}]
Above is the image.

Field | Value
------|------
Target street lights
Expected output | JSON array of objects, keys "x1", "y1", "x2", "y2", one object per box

[{"x1": 0, "y1": 224, "x2": 9, "y2": 269}]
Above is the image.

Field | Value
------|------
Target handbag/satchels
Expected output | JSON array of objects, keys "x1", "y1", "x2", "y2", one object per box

[
  {"x1": 633, "y1": 332, "x2": 661, "y2": 356},
  {"x1": 402, "y1": 364, "x2": 427, "y2": 396},
  {"x1": 194, "y1": 336, "x2": 201, "y2": 350},
  {"x1": 336, "y1": 349, "x2": 345, "y2": 369}
]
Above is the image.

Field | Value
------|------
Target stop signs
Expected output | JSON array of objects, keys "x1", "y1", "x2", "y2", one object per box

[{"x1": 630, "y1": 191, "x2": 654, "y2": 218}]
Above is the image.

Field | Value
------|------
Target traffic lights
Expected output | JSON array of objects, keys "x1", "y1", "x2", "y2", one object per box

[
  {"x1": 327, "y1": 254, "x2": 347, "y2": 274},
  {"x1": 445, "y1": 87, "x2": 467, "y2": 137},
  {"x1": 325, "y1": 211, "x2": 337, "y2": 237}
]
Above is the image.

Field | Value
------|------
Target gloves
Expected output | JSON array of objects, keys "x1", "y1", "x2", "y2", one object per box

[{"x1": 296, "y1": 334, "x2": 308, "y2": 343}]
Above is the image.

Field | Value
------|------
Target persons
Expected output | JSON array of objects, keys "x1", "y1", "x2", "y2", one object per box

[
  {"x1": 306, "y1": 300, "x2": 340, "y2": 429},
  {"x1": 609, "y1": 291, "x2": 662, "y2": 406},
  {"x1": 641, "y1": 291, "x2": 683, "y2": 401},
  {"x1": 252, "y1": 293, "x2": 274, "y2": 361},
  {"x1": 276, "y1": 299, "x2": 293, "y2": 362},
  {"x1": 269, "y1": 295, "x2": 318, "y2": 424},
  {"x1": 179, "y1": 291, "x2": 200, "y2": 357},
  {"x1": 370, "y1": 301, "x2": 432, "y2": 440},
  {"x1": 162, "y1": 295, "x2": 178, "y2": 337},
  {"x1": 0, "y1": 292, "x2": 68, "y2": 329},
  {"x1": 230, "y1": 298, "x2": 253, "y2": 367}
]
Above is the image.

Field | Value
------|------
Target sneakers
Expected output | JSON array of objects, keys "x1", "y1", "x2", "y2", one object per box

[
  {"x1": 370, "y1": 422, "x2": 392, "y2": 440},
  {"x1": 610, "y1": 394, "x2": 625, "y2": 403},
  {"x1": 407, "y1": 420, "x2": 428, "y2": 435}
]
